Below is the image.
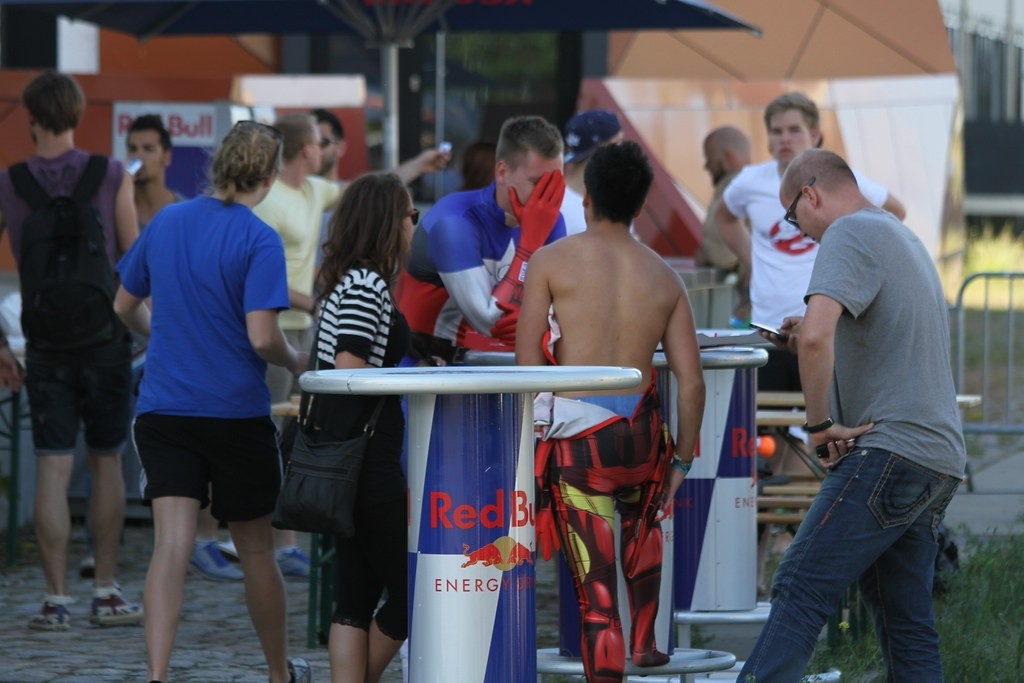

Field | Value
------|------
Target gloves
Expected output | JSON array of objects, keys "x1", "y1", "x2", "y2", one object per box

[
  {"x1": 492, "y1": 302, "x2": 521, "y2": 346},
  {"x1": 492, "y1": 168, "x2": 564, "y2": 308}
]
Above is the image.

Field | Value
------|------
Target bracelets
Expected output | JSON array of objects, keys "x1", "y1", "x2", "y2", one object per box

[
  {"x1": 668, "y1": 447, "x2": 695, "y2": 475},
  {"x1": 802, "y1": 416, "x2": 836, "y2": 433}
]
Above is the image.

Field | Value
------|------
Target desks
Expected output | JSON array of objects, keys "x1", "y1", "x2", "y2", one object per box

[{"x1": 245, "y1": 394, "x2": 983, "y2": 648}]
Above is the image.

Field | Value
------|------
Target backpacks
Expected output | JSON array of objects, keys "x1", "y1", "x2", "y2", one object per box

[{"x1": 7, "y1": 153, "x2": 125, "y2": 351}]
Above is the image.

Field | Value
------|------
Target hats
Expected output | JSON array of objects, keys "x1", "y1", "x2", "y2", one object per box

[{"x1": 562, "y1": 110, "x2": 622, "y2": 165}]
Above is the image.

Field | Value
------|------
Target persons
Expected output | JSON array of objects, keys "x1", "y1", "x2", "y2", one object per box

[
  {"x1": 301, "y1": 170, "x2": 457, "y2": 683},
  {"x1": 732, "y1": 147, "x2": 967, "y2": 683},
  {"x1": 0, "y1": 89, "x2": 908, "y2": 581},
  {"x1": 0, "y1": 66, "x2": 152, "y2": 631},
  {"x1": 514, "y1": 138, "x2": 708, "y2": 683},
  {"x1": 109, "y1": 119, "x2": 316, "y2": 683}
]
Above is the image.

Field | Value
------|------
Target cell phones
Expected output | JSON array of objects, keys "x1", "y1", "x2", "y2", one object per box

[{"x1": 750, "y1": 322, "x2": 786, "y2": 341}]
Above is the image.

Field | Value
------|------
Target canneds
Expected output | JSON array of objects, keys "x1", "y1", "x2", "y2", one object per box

[{"x1": 124, "y1": 157, "x2": 143, "y2": 176}]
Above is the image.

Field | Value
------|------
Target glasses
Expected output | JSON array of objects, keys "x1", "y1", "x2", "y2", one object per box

[
  {"x1": 403, "y1": 209, "x2": 420, "y2": 224},
  {"x1": 320, "y1": 137, "x2": 338, "y2": 147},
  {"x1": 781, "y1": 177, "x2": 818, "y2": 228},
  {"x1": 223, "y1": 121, "x2": 282, "y2": 175}
]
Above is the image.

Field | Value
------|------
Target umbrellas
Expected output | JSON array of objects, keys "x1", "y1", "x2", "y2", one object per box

[{"x1": 0, "y1": 0, "x2": 766, "y2": 207}]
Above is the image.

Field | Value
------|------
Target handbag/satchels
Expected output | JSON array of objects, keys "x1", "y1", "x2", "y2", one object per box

[{"x1": 271, "y1": 423, "x2": 371, "y2": 541}]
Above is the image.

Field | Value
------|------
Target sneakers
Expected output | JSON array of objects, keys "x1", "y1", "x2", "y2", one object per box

[
  {"x1": 28, "y1": 601, "x2": 71, "y2": 630},
  {"x1": 268, "y1": 657, "x2": 312, "y2": 682},
  {"x1": 190, "y1": 541, "x2": 246, "y2": 583},
  {"x1": 276, "y1": 550, "x2": 312, "y2": 578},
  {"x1": 90, "y1": 595, "x2": 144, "y2": 626}
]
